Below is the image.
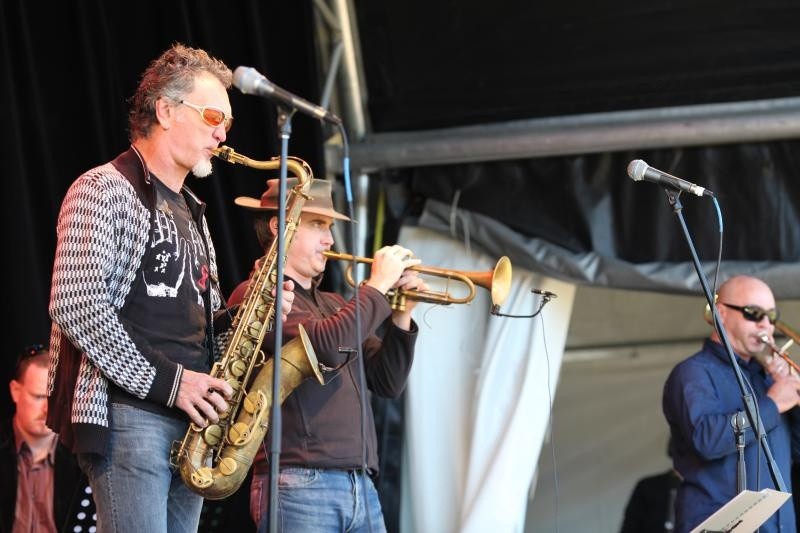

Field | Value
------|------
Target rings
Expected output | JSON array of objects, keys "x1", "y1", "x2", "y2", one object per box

[{"x1": 777, "y1": 365, "x2": 783, "y2": 372}]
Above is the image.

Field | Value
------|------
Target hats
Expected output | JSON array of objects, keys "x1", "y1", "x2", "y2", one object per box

[{"x1": 234, "y1": 176, "x2": 350, "y2": 220}]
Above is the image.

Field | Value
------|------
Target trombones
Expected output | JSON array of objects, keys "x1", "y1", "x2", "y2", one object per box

[{"x1": 704, "y1": 293, "x2": 800, "y2": 400}]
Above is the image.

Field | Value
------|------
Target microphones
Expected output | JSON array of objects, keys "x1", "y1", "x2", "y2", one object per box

[
  {"x1": 532, "y1": 289, "x2": 557, "y2": 298},
  {"x1": 232, "y1": 65, "x2": 342, "y2": 125},
  {"x1": 627, "y1": 159, "x2": 713, "y2": 199},
  {"x1": 338, "y1": 347, "x2": 357, "y2": 352}
]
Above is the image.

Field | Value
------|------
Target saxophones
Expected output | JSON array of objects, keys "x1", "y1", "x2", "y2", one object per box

[{"x1": 175, "y1": 145, "x2": 325, "y2": 498}]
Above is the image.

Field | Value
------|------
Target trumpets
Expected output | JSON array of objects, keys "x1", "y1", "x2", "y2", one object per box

[{"x1": 322, "y1": 249, "x2": 513, "y2": 314}]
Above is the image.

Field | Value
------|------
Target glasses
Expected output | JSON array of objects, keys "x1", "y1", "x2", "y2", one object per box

[
  {"x1": 174, "y1": 101, "x2": 233, "y2": 134},
  {"x1": 720, "y1": 302, "x2": 778, "y2": 324}
]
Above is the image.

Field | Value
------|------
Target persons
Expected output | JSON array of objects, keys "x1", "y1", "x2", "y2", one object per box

[
  {"x1": 228, "y1": 177, "x2": 429, "y2": 533},
  {"x1": 0, "y1": 352, "x2": 81, "y2": 533},
  {"x1": 46, "y1": 47, "x2": 293, "y2": 533},
  {"x1": 662, "y1": 275, "x2": 800, "y2": 533}
]
